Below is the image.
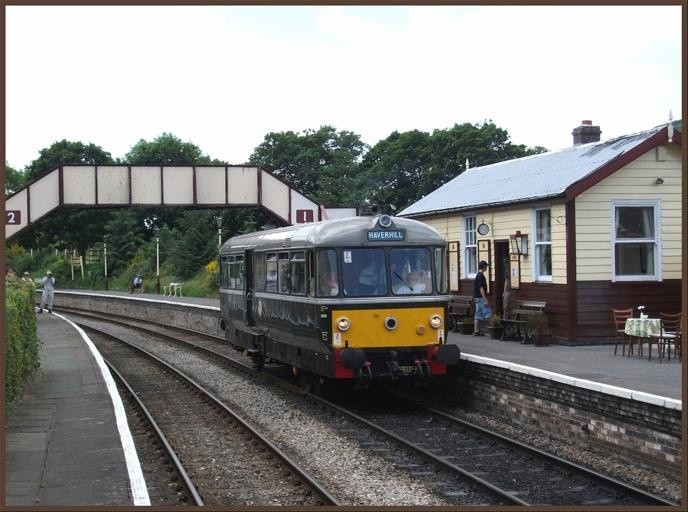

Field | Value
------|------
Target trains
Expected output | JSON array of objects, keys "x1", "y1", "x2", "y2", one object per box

[{"x1": 217, "y1": 215, "x2": 461, "y2": 400}]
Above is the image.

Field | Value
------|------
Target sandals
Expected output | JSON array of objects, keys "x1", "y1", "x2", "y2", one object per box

[{"x1": 474, "y1": 330, "x2": 485, "y2": 336}]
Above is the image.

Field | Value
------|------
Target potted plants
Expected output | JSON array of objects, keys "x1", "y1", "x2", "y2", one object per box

[
  {"x1": 528, "y1": 311, "x2": 551, "y2": 347},
  {"x1": 486, "y1": 315, "x2": 503, "y2": 338},
  {"x1": 457, "y1": 316, "x2": 475, "y2": 334}
]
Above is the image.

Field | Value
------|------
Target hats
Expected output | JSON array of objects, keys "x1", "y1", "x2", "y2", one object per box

[{"x1": 479, "y1": 261, "x2": 489, "y2": 269}]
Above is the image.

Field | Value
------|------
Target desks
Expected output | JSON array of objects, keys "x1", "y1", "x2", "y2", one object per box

[{"x1": 623, "y1": 317, "x2": 666, "y2": 362}]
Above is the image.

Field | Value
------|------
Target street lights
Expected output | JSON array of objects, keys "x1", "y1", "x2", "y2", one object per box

[
  {"x1": 102, "y1": 235, "x2": 109, "y2": 291},
  {"x1": 217, "y1": 217, "x2": 224, "y2": 254},
  {"x1": 155, "y1": 226, "x2": 162, "y2": 293}
]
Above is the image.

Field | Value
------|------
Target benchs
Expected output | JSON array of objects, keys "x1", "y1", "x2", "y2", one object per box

[
  {"x1": 447, "y1": 295, "x2": 475, "y2": 332},
  {"x1": 162, "y1": 282, "x2": 183, "y2": 297},
  {"x1": 134, "y1": 282, "x2": 144, "y2": 294},
  {"x1": 497, "y1": 298, "x2": 547, "y2": 344}
]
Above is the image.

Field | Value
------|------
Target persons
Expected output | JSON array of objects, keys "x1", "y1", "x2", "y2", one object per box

[
  {"x1": 21, "y1": 272, "x2": 35, "y2": 286},
  {"x1": 129, "y1": 275, "x2": 142, "y2": 293},
  {"x1": 323, "y1": 258, "x2": 431, "y2": 296},
  {"x1": 502, "y1": 258, "x2": 511, "y2": 319},
  {"x1": 472, "y1": 260, "x2": 493, "y2": 337},
  {"x1": 38, "y1": 270, "x2": 56, "y2": 314}
]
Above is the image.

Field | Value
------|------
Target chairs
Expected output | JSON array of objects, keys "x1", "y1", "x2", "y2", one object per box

[
  {"x1": 611, "y1": 308, "x2": 642, "y2": 357},
  {"x1": 647, "y1": 311, "x2": 681, "y2": 363}
]
